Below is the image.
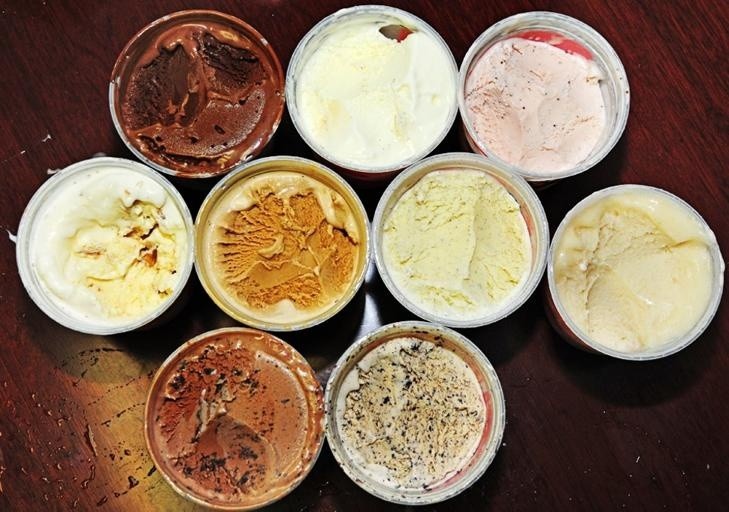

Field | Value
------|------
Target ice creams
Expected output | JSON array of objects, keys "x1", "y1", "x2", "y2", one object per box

[{"x1": 16, "y1": 5, "x2": 722, "y2": 511}]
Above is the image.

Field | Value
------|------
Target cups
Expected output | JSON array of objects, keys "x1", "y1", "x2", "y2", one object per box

[
  {"x1": 143, "y1": 326, "x2": 325, "y2": 508},
  {"x1": 109, "y1": 9, "x2": 283, "y2": 180},
  {"x1": 15, "y1": 156, "x2": 192, "y2": 340},
  {"x1": 456, "y1": 11, "x2": 630, "y2": 183},
  {"x1": 322, "y1": 319, "x2": 506, "y2": 503},
  {"x1": 195, "y1": 156, "x2": 371, "y2": 334},
  {"x1": 371, "y1": 152, "x2": 551, "y2": 327},
  {"x1": 283, "y1": 6, "x2": 460, "y2": 175},
  {"x1": 552, "y1": 186, "x2": 725, "y2": 361}
]
